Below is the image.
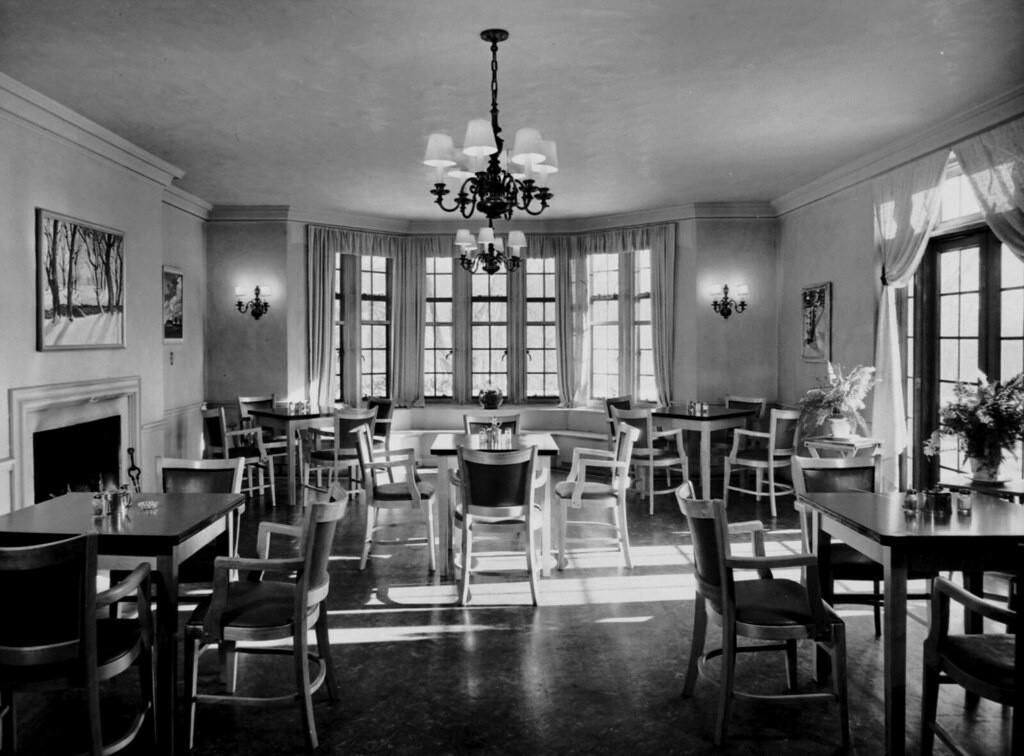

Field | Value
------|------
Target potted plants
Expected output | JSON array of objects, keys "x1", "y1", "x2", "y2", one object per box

[{"x1": 789, "y1": 361, "x2": 884, "y2": 441}]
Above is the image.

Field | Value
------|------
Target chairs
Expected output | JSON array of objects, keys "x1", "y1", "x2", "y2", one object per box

[
  {"x1": 182, "y1": 482, "x2": 348, "y2": 751},
  {"x1": 606, "y1": 395, "x2": 1024, "y2": 756},
  {"x1": 1, "y1": 531, "x2": 158, "y2": 756},
  {"x1": 350, "y1": 423, "x2": 439, "y2": 572},
  {"x1": 554, "y1": 422, "x2": 641, "y2": 572},
  {"x1": 448, "y1": 442, "x2": 539, "y2": 609},
  {"x1": 201, "y1": 392, "x2": 522, "y2": 510},
  {"x1": 108, "y1": 457, "x2": 243, "y2": 632}
]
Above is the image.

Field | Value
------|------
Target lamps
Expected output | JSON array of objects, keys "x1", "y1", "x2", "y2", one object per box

[
  {"x1": 234, "y1": 285, "x2": 272, "y2": 321},
  {"x1": 423, "y1": 29, "x2": 558, "y2": 221},
  {"x1": 455, "y1": 217, "x2": 528, "y2": 276},
  {"x1": 711, "y1": 283, "x2": 748, "y2": 319}
]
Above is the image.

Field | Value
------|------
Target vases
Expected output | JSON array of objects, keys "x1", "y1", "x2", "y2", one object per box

[{"x1": 970, "y1": 448, "x2": 1012, "y2": 483}]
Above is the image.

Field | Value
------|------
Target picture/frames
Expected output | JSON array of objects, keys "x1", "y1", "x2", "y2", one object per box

[
  {"x1": 162, "y1": 265, "x2": 184, "y2": 345},
  {"x1": 800, "y1": 280, "x2": 833, "y2": 363},
  {"x1": 36, "y1": 207, "x2": 127, "y2": 351}
]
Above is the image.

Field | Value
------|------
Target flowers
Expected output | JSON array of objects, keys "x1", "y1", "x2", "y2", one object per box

[{"x1": 922, "y1": 366, "x2": 1024, "y2": 475}]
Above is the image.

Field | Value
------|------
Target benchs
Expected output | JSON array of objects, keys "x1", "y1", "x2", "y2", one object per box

[{"x1": 385, "y1": 410, "x2": 620, "y2": 469}]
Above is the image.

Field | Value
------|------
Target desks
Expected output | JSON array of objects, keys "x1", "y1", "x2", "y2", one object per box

[
  {"x1": 938, "y1": 480, "x2": 1024, "y2": 506},
  {"x1": 797, "y1": 490, "x2": 1024, "y2": 756},
  {"x1": 804, "y1": 435, "x2": 882, "y2": 459},
  {"x1": 652, "y1": 405, "x2": 756, "y2": 500},
  {"x1": 247, "y1": 407, "x2": 335, "y2": 507},
  {"x1": 430, "y1": 432, "x2": 559, "y2": 579},
  {"x1": 0, "y1": 491, "x2": 246, "y2": 756}
]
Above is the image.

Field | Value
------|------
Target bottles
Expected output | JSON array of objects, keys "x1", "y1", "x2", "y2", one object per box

[
  {"x1": 92, "y1": 495, "x2": 104, "y2": 517},
  {"x1": 479, "y1": 427, "x2": 486, "y2": 444},
  {"x1": 101, "y1": 488, "x2": 112, "y2": 514},
  {"x1": 688, "y1": 400, "x2": 695, "y2": 415},
  {"x1": 958, "y1": 489, "x2": 972, "y2": 510},
  {"x1": 305, "y1": 401, "x2": 312, "y2": 415},
  {"x1": 289, "y1": 402, "x2": 295, "y2": 414},
  {"x1": 504, "y1": 428, "x2": 512, "y2": 445},
  {"x1": 702, "y1": 402, "x2": 710, "y2": 417},
  {"x1": 905, "y1": 489, "x2": 918, "y2": 516}
]
